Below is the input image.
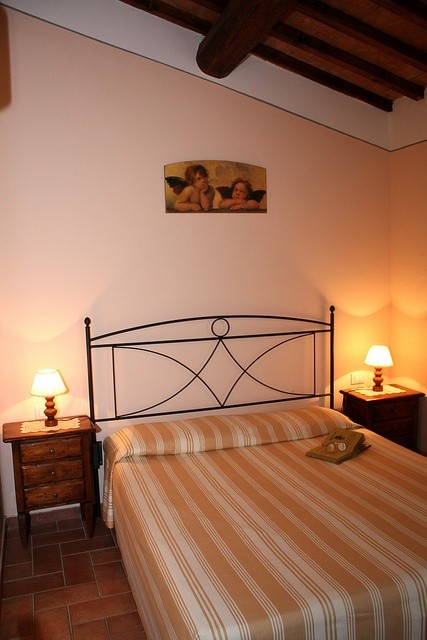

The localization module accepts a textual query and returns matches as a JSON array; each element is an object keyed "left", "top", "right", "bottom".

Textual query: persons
[
  {"left": 164, "top": 164, "right": 215, "bottom": 212},
  {"left": 214, "top": 177, "right": 267, "bottom": 212}
]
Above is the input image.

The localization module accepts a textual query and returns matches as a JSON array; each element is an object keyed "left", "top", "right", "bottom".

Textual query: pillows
[{"left": 124, "top": 403, "right": 353, "bottom": 456}]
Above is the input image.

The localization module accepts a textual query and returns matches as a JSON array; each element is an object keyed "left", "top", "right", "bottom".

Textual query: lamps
[
  {"left": 29, "top": 368, "right": 68, "bottom": 428},
  {"left": 365, "top": 345, "right": 395, "bottom": 392}
]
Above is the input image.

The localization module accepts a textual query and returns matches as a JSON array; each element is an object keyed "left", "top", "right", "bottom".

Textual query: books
[{"left": 303, "top": 427, "right": 369, "bottom": 464}]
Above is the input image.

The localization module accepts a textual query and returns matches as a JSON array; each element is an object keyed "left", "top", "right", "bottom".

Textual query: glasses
[{"left": 321, "top": 442, "right": 347, "bottom": 452}]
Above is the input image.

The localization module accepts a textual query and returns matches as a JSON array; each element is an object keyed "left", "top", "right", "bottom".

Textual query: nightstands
[
  {"left": 339, "top": 384, "right": 425, "bottom": 453},
  {"left": 2, "top": 415, "right": 96, "bottom": 547}
]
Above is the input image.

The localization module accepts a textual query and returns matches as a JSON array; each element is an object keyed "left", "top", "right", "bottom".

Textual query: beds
[{"left": 85, "top": 305, "right": 426, "bottom": 640}]
